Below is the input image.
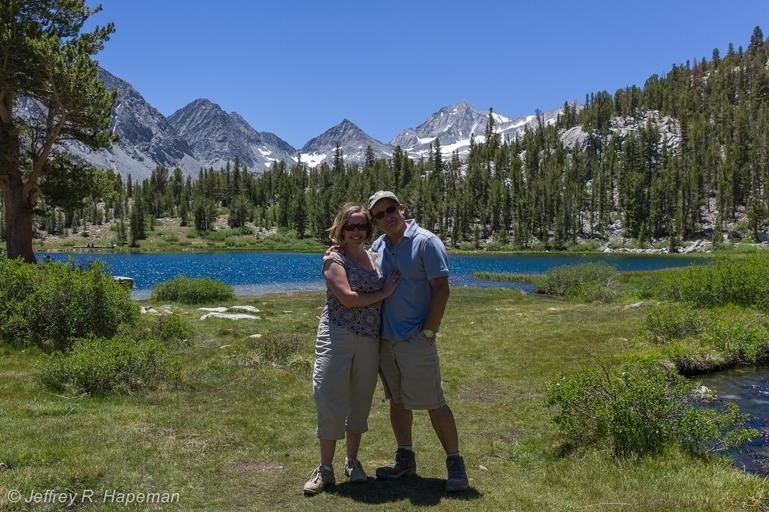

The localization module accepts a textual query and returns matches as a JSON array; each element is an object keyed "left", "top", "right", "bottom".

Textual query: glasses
[
  {"left": 344, "top": 222, "right": 368, "bottom": 232},
  {"left": 371, "top": 203, "right": 398, "bottom": 218}
]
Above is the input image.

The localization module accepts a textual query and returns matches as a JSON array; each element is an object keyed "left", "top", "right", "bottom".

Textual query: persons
[
  {"left": 303, "top": 201, "right": 402, "bottom": 495},
  {"left": 324, "top": 191, "right": 468, "bottom": 492}
]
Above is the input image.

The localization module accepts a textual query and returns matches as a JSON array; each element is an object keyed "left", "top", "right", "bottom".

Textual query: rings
[{"left": 392, "top": 279, "right": 396, "bottom": 283}]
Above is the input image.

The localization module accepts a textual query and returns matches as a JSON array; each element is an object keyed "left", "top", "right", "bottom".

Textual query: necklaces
[{"left": 357, "top": 260, "right": 361, "bottom": 267}]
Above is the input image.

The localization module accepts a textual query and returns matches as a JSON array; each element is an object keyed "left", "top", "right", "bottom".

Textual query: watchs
[{"left": 424, "top": 328, "right": 437, "bottom": 341}]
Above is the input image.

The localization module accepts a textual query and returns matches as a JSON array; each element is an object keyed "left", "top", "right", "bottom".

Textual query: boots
[
  {"left": 444, "top": 454, "right": 470, "bottom": 491},
  {"left": 376, "top": 449, "right": 417, "bottom": 479}
]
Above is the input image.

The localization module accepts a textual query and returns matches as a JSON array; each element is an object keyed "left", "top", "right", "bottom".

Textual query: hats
[{"left": 367, "top": 190, "right": 402, "bottom": 208}]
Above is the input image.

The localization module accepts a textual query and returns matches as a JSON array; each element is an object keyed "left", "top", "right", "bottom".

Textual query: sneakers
[
  {"left": 344, "top": 458, "right": 369, "bottom": 483},
  {"left": 305, "top": 465, "right": 336, "bottom": 494}
]
